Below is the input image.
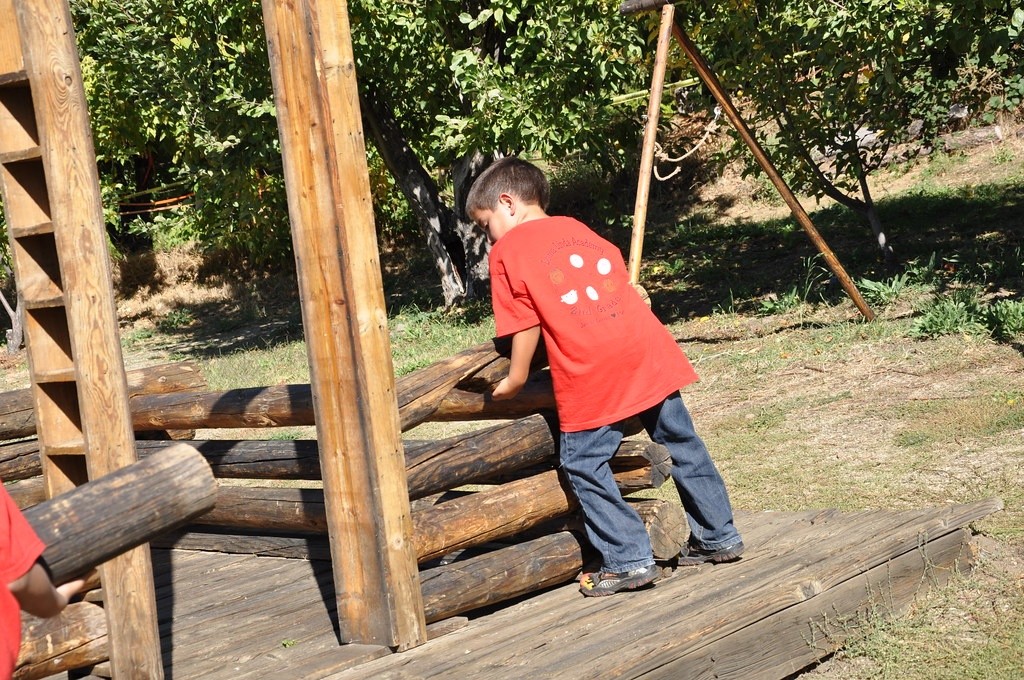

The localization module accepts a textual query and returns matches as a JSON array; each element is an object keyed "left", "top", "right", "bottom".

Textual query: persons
[
  {"left": 463, "top": 156, "right": 744, "bottom": 597},
  {"left": 0, "top": 481, "right": 87, "bottom": 680}
]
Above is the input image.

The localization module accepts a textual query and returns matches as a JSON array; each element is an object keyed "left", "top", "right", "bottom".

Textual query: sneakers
[
  {"left": 678, "top": 541, "right": 745, "bottom": 566},
  {"left": 580, "top": 564, "right": 658, "bottom": 597}
]
